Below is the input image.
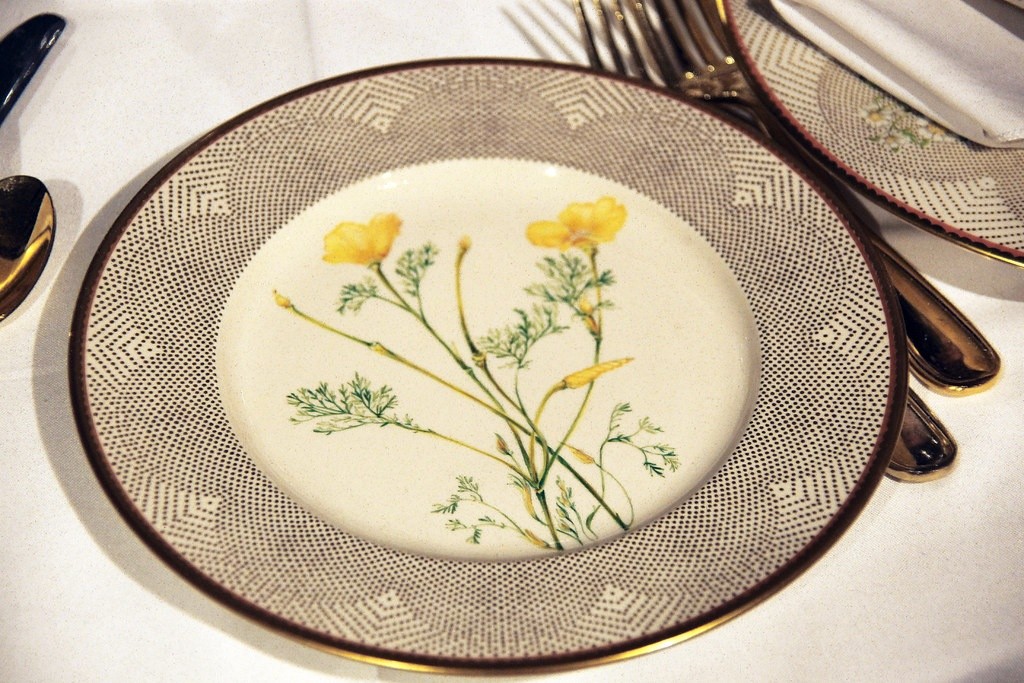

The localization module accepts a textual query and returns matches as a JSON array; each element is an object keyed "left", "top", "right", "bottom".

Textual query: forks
[{"left": 578, "top": 1, "right": 1000, "bottom": 485}]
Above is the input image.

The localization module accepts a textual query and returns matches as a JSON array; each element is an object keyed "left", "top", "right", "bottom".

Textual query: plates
[
  {"left": 722, "top": 3, "right": 1024, "bottom": 266},
  {"left": 65, "top": 58, "right": 912, "bottom": 683}
]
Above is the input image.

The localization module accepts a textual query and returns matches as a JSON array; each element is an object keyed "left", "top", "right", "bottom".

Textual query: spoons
[{"left": 0, "top": 176, "right": 56, "bottom": 323}]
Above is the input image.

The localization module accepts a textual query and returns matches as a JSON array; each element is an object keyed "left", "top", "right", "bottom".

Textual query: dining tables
[{"left": 1, "top": 0, "right": 1019, "bottom": 683}]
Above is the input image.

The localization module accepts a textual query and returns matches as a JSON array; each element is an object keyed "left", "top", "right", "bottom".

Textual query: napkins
[{"left": 770, "top": 0, "right": 1022, "bottom": 150}]
